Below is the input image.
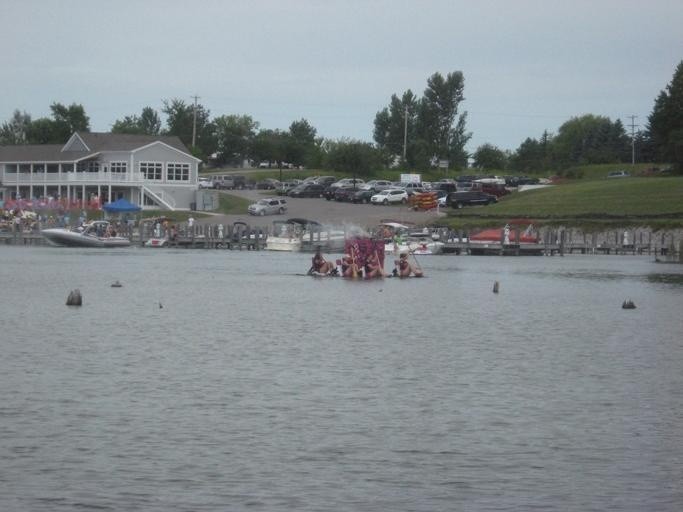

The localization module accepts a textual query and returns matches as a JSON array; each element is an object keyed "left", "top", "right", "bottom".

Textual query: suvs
[{"left": 604, "top": 170, "right": 630, "bottom": 177}]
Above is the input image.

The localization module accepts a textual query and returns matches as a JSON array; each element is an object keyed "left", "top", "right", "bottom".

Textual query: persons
[
  {"left": 0, "top": 193, "right": 196, "bottom": 241},
  {"left": 311, "top": 225, "right": 423, "bottom": 281}
]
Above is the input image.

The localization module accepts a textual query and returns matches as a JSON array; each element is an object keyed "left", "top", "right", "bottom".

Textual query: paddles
[
  {"left": 350, "top": 247, "right": 358, "bottom": 276},
  {"left": 375, "top": 249, "right": 384, "bottom": 276}
]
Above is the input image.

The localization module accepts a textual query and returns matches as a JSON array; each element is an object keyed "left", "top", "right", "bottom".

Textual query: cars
[{"left": 661, "top": 164, "right": 678, "bottom": 172}]
